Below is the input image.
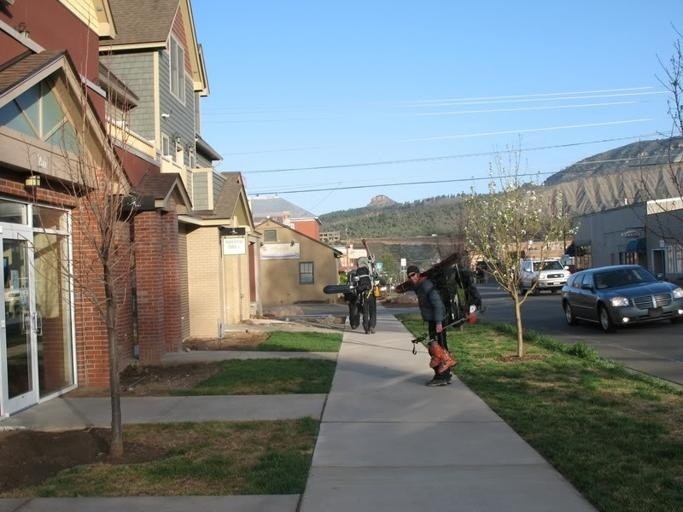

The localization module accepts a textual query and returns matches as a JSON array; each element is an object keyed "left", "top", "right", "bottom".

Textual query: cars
[{"left": 560, "top": 262, "right": 682, "bottom": 333}]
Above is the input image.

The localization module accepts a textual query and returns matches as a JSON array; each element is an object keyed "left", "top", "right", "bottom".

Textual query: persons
[
  {"left": 357, "top": 261, "right": 381, "bottom": 335},
  {"left": 343, "top": 261, "right": 361, "bottom": 330},
  {"left": 406, "top": 264, "right": 453, "bottom": 387}
]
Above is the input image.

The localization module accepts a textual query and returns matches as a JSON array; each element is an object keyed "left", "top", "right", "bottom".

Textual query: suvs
[{"left": 511, "top": 257, "right": 571, "bottom": 294}]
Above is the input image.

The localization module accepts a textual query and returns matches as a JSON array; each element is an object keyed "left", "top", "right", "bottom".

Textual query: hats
[{"left": 407, "top": 266, "right": 420, "bottom": 275}]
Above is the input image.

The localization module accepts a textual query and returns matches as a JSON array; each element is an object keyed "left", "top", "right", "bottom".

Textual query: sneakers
[
  {"left": 425, "top": 377, "right": 452, "bottom": 387},
  {"left": 351, "top": 325, "right": 375, "bottom": 335}
]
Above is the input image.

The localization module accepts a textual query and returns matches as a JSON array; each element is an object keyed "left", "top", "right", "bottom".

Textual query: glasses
[{"left": 408, "top": 272, "right": 416, "bottom": 279}]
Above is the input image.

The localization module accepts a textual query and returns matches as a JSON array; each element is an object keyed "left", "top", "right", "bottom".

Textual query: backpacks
[
  {"left": 427, "top": 263, "right": 483, "bottom": 330},
  {"left": 355, "top": 267, "right": 372, "bottom": 293}
]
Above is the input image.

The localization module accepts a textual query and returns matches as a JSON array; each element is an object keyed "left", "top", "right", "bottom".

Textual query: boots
[
  {"left": 437, "top": 346, "right": 457, "bottom": 375},
  {"left": 427, "top": 339, "right": 448, "bottom": 368}
]
